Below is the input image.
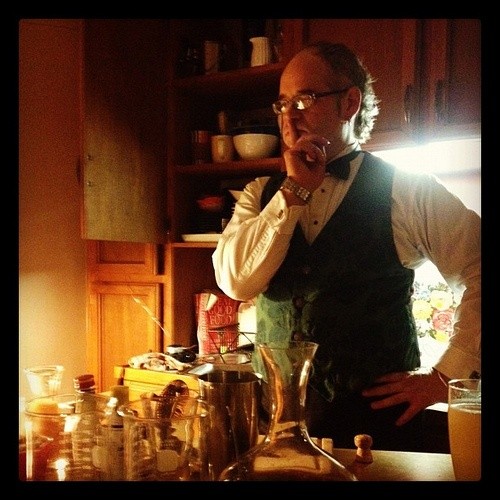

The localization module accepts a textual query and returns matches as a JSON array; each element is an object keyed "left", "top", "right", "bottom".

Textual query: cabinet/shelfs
[
  {"left": 80, "top": 210, "right": 218, "bottom": 422},
  {"left": 76, "top": 16, "right": 304, "bottom": 251},
  {"left": 277, "top": 18, "right": 482, "bottom": 154}
]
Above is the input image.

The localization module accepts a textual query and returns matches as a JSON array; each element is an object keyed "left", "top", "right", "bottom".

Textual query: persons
[{"left": 211, "top": 41, "right": 481, "bottom": 452}]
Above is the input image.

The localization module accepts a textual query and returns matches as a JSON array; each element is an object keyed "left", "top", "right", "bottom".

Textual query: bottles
[
  {"left": 218, "top": 337, "right": 360, "bottom": 479},
  {"left": 249, "top": 33, "right": 274, "bottom": 66}
]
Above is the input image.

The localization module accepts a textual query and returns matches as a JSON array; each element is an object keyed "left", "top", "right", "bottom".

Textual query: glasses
[{"left": 272, "top": 88, "right": 348, "bottom": 115}]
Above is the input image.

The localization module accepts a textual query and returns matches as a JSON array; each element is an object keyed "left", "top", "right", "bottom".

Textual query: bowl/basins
[
  {"left": 196, "top": 194, "right": 224, "bottom": 212},
  {"left": 233, "top": 133, "right": 278, "bottom": 161}
]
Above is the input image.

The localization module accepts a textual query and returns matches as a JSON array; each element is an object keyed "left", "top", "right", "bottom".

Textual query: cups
[
  {"left": 25, "top": 360, "right": 260, "bottom": 481},
  {"left": 210, "top": 134, "right": 233, "bottom": 161},
  {"left": 447, "top": 374, "right": 480, "bottom": 481}
]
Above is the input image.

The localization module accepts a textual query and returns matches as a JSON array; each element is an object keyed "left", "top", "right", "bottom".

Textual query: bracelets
[
  {"left": 281, "top": 177, "right": 313, "bottom": 204},
  {"left": 438, "top": 370, "right": 449, "bottom": 390}
]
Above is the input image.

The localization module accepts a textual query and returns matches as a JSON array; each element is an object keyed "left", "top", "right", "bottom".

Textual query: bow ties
[{"left": 325, "top": 156, "right": 351, "bottom": 180}]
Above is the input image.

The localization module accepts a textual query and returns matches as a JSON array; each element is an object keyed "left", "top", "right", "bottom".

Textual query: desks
[{"left": 19, "top": 435, "right": 457, "bottom": 482}]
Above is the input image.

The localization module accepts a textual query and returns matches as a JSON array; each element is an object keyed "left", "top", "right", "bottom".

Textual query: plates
[{"left": 181, "top": 230, "right": 223, "bottom": 243}]
